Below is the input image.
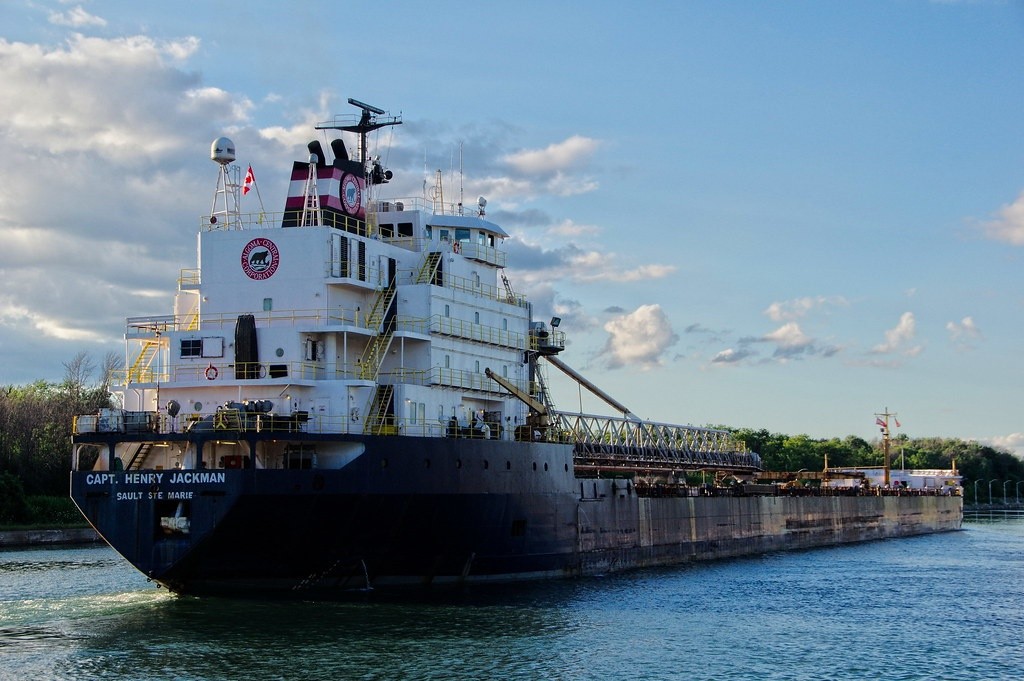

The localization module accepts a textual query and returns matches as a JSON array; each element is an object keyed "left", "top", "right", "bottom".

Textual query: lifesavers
[
  {"left": 453, "top": 242, "right": 459, "bottom": 254},
  {"left": 204, "top": 367, "right": 219, "bottom": 380}
]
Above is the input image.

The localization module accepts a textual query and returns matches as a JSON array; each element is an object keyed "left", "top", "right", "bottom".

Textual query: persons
[{"left": 481, "top": 420, "right": 491, "bottom": 439}]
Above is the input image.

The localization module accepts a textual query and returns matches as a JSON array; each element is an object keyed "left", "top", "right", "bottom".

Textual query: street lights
[{"left": 973, "top": 480, "right": 1024, "bottom": 505}]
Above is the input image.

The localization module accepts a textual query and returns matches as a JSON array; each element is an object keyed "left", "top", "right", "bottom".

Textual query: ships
[{"left": 69, "top": 95, "right": 963, "bottom": 607}]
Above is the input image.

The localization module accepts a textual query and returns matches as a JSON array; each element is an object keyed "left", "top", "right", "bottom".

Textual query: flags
[
  {"left": 876, "top": 418, "right": 887, "bottom": 429},
  {"left": 243, "top": 167, "right": 255, "bottom": 196}
]
